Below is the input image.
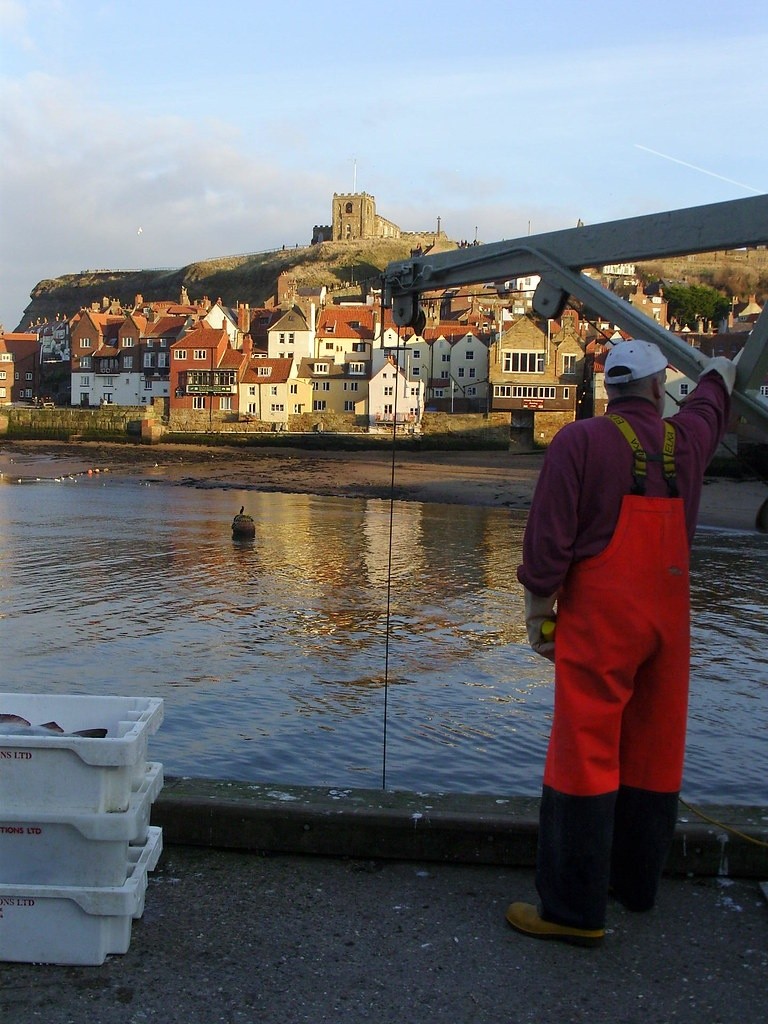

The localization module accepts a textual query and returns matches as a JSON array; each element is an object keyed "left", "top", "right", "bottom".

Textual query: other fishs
[{"left": 0, "top": 712, "right": 108, "bottom": 744}]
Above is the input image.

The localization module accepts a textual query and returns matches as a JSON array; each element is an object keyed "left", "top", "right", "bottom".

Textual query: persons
[{"left": 506, "top": 339, "right": 737, "bottom": 947}]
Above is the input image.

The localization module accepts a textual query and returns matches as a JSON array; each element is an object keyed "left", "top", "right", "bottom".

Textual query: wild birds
[{"left": 9, "top": 456, "right": 159, "bottom": 486}]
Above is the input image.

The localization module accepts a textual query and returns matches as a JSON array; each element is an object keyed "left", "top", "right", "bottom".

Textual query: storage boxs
[{"left": 0, "top": 692, "right": 164, "bottom": 968}]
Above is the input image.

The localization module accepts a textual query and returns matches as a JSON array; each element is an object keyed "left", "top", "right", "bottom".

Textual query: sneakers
[{"left": 504, "top": 901, "right": 605, "bottom": 950}]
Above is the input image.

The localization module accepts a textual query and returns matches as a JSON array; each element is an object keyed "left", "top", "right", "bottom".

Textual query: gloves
[
  {"left": 524, "top": 584, "right": 560, "bottom": 665},
  {"left": 699, "top": 356, "right": 736, "bottom": 396}
]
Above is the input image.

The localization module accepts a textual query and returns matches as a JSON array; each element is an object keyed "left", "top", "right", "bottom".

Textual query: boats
[{"left": 233, "top": 504, "right": 256, "bottom": 538}]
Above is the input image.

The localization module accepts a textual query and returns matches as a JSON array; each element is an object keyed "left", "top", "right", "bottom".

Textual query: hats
[{"left": 604, "top": 339, "right": 678, "bottom": 386}]
